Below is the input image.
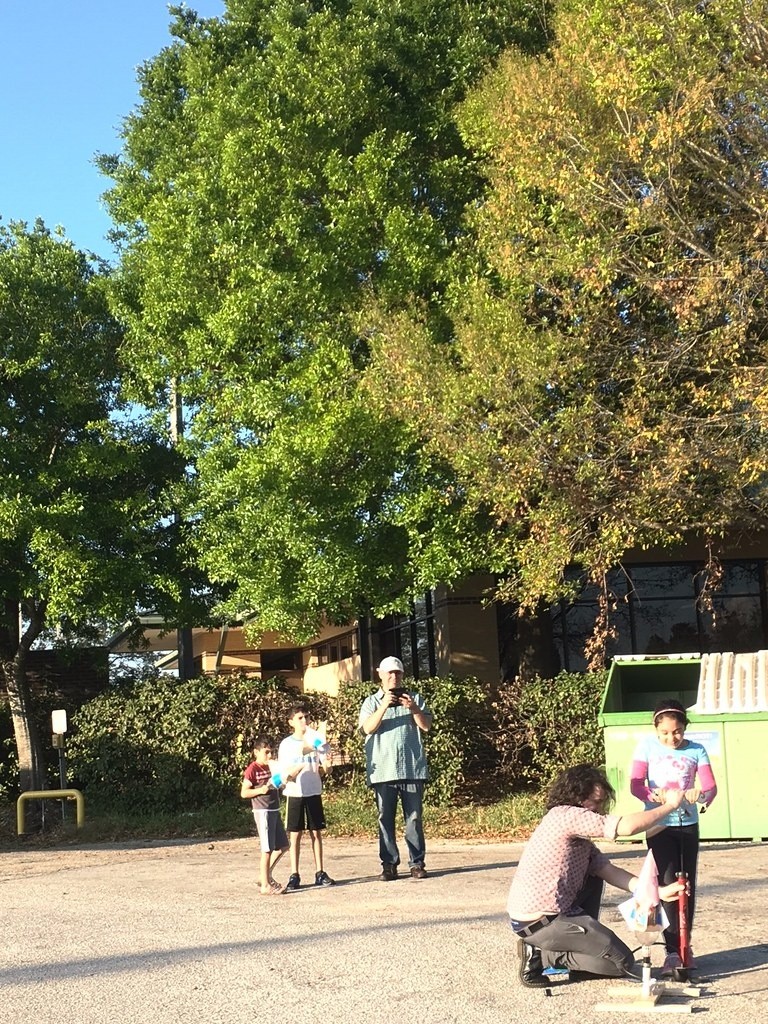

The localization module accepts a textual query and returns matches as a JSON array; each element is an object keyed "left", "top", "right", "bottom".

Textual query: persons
[
  {"left": 630, "top": 703, "right": 718, "bottom": 975},
  {"left": 357, "top": 656, "right": 431, "bottom": 880},
  {"left": 241, "top": 736, "right": 289, "bottom": 894},
  {"left": 277, "top": 703, "right": 335, "bottom": 890},
  {"left": 507, "top": 764, "right": 683, "bottom": 988}
]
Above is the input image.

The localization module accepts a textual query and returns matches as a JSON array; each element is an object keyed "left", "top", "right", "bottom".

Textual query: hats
[{"left": 379, "top": 656, "right": 405, "bottom": 673}]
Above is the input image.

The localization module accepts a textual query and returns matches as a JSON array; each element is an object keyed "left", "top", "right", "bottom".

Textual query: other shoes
[
  {"left": 316, "top": 871, "right": 336, "bottom": 886},
  {"left": 286, "top": 876, "right": 299, "bottom": 889},
  {"left": 411, "top": 868, "right": 427, "bottom": 877},
  {"left": 659, "top": 951, "right": 683, "bottom": 976},
  {"left": 517, "top": 938, "right": 550, "bottom": 988},
  {"left": 568, "top": 970, "right": 627, "bottom": 982},
  {"left": 379, "top": 870, "right": 398, "bottom": 881},
  {"left": 680, "top": 947, "right": 698, "bottom": 970},
  {"left": 259, "top": 880, "right": 286, "bottom": 894}
]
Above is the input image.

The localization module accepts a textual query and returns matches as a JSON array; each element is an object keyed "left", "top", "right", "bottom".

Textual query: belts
[{"left": 516, "top": 915, "right": 558, "bottom": 938}]
